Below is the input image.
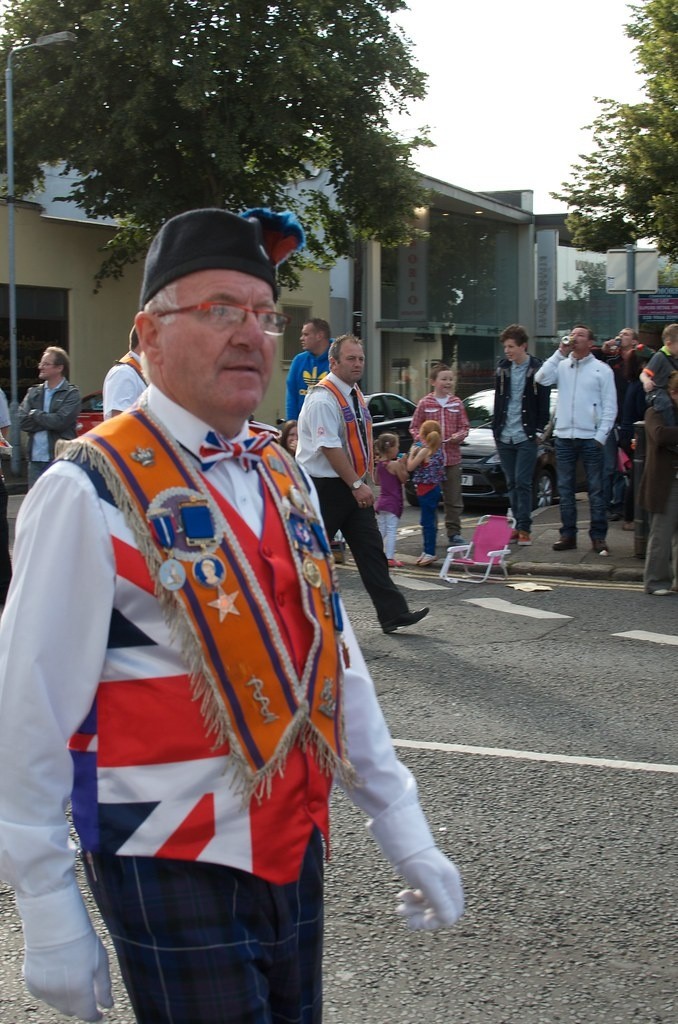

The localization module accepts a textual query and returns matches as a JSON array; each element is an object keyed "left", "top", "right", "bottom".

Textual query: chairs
[
  {"left": 437, "top": 514, "right": 517, "bottom": 584},
  {"left": 370, "top": 404, "right": 383, "bottom": 416}
]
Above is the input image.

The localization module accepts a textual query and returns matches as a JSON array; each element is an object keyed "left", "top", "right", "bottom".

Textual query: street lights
[{"left": 5, "top": 31, "right": 77, "bottom": 478}]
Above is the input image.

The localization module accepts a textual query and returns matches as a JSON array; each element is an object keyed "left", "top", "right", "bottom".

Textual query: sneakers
[
  {"left": 449, "top": 534, "right": 466, "bottom": 545},
  {"left": 517, "top": 531, "right": 533, "bottom": 546},
  {"left": 624, "top": 521, "right": 637, "bottom": 531},
  {"left": 553, "top": 536, "right": 577, "bottom": 550},
  {"left": 593, "top": 539, "right": 608, "bottom": 553},
  {"left": 509, "top": 529, "right": 517, "bottom": 544}
]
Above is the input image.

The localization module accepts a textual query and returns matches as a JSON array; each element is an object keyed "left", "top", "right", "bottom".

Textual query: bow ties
[{"left": 199, "top": 431, "right": 271, "bottom": 473}]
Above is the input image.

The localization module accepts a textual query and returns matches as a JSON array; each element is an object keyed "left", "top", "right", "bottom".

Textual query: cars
[
  {"left": 77, "top": 387, "right": 105, "bottom": 439},
  {"left": 363, "top": 392, "right": 418, "bottom": 456},
  {"left": 405, "top": 387, "right": 582, "bottom": 511}
]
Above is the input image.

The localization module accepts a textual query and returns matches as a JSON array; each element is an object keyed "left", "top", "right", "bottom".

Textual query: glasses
[
  {"left": 39, "top": 362, "right": 61, "bottom": 368},
  {"left": 157, "top": 302, "right": 288, "bottom": 336}
]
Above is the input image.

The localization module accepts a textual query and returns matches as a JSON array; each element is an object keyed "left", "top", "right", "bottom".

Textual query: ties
[{"left": 350, "top": 389, "right": 368, "bottom": 456}]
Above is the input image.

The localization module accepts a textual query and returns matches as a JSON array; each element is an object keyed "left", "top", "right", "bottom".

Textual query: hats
[{"left": 138, "top": 208, "right": 302, "bottom": 309}]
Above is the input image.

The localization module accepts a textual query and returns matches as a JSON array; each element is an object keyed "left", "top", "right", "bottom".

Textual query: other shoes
[
  {"left": 651, "top": 588, "right": 676, "bottom": 595},
  {"left": 387, "top": 559, "right": 402, "bottom": 566},
  {"left": 416, "top": 553, "right": 425, "bottom": 564},
  {"left": 419, "top": 554, "right": 438, "bottom": 565}
]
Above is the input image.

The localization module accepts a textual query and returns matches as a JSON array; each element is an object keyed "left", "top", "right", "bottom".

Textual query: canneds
[{"left": 561, "top": 336, "right": 575, "bottom": 345}]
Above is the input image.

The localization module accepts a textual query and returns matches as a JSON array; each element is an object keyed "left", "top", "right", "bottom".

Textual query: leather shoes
[{"left": 381, "top": 607, "right": 429, "bottom": 634}]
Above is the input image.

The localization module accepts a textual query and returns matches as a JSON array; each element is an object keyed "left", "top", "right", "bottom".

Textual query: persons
[
  {"left": 102, "top": 325, "right": 150, "bottom": 420},
  {"left": 294, "top": 334, "right": 430, "bottom": 633},
  {"left": 280, "top": 419, "right": 298, "bottom": 456},
  {"left": 286, "top": 318, "right": 337, "bottom": 421},
  {"left": 0, "top": 208, "right": 463, "bottom": 1024},
  {"left": 409, "top": 364, "right": 469, "bottom": 545},
  {"left": 534, "top": 325, "right": 618, "bottom": 556},
  {"left": 17, "top": 347, "right": 81, "bottom": 490},
  {"left": 407, "top": 420, "right": 447, "bottom": 565},
  {"left": 376, "top": 434, "right": 407, "bottom": 567},
  {"left": 0, "top": 389, "right": 11, "bottom": 438},
  {"left": 590, "top": 324, "right": 678, "bottom": 595},
  {"left": 492, "top": 324, "right": 550, "bottom": 545}
]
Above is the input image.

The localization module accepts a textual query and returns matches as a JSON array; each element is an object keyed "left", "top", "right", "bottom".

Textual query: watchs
[{"left": 350, "top": 479, "right": 361, "bottom": 490}]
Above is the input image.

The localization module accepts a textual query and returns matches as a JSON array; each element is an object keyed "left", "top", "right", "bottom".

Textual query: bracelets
[
  {"left": 29, "top": 410, "right": 35, "bottom": 415},
  {"left": 536, "top": 433, "right": 543, "bottom": 439}
]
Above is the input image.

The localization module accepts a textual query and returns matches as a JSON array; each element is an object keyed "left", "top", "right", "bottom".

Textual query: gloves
[
  {"left": 394, "top": 848, "right": 463, "bottom": 932},
  {"left": 16, "top": 886, "right": 114, "bottom": 1021}
]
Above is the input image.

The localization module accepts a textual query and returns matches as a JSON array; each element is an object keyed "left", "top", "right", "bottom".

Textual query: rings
[{"left": 363, "top": 502, "right": 366, "bottom": 504}]
